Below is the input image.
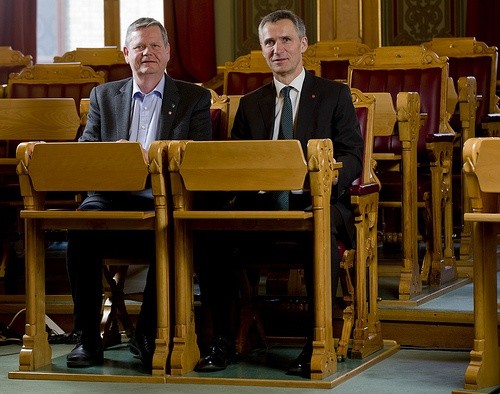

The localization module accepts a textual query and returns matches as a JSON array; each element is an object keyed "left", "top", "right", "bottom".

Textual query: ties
[{"left": 273, "top": 86, "right": 294, "bottom": 211}]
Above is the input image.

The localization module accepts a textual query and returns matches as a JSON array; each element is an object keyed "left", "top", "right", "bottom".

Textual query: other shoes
[
  {"left": 199, "top": 338, "right": 241, "bottom": 372},
  {"left": 129, "top": 333, "right": 155, "bottom": 366},
  {"left": 296, "top": 346, "right": 311, "bottom": 375},
  {"left": 67, "top": 338, "right": 104, "bottom": 368}
]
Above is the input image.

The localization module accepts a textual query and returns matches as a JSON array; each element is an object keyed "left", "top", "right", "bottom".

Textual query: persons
[
  {"left": 194, "top": 11, "right": 364, "bottom": 373},
  {"left": 66, "top": 18, "right": 211, "bottom": 370}
]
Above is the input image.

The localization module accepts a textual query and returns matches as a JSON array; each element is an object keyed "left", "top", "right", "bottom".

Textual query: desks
[{"left": 10, "top": 138, "right": 500, "bottom": 394}]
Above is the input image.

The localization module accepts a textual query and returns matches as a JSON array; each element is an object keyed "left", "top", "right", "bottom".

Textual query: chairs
[{"left": 1, "top": 37, "right": 500, "bottom": 356}]
